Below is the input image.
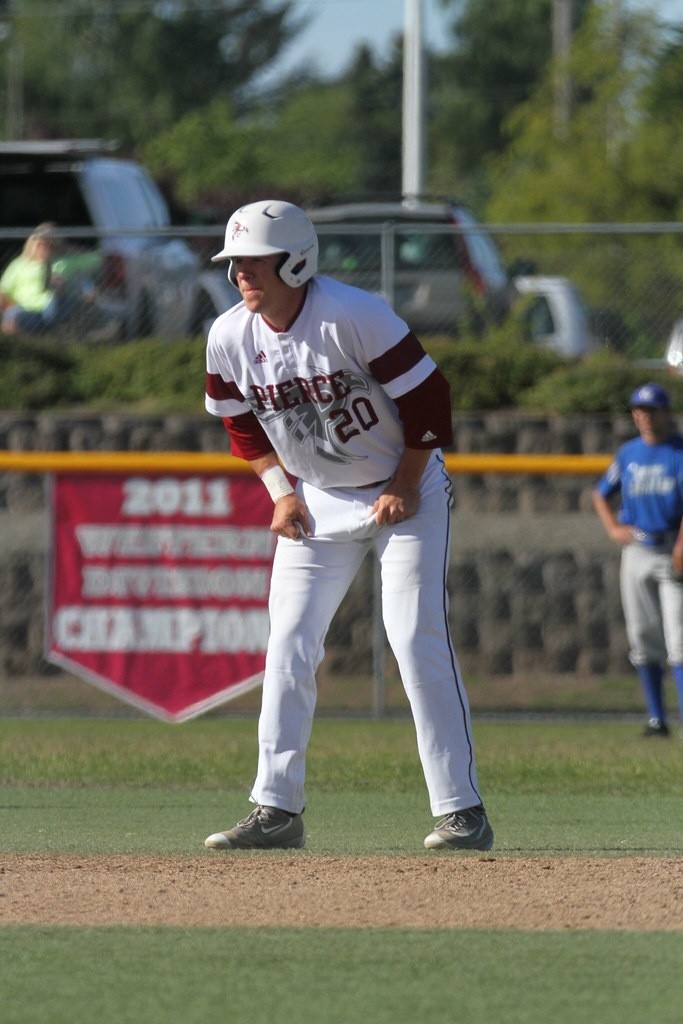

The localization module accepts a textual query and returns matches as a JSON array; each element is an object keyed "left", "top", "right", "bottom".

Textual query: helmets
[
  {"left": 630, "top": 384, "right": 670, "bottom": 410},
  {"left": 211, "top": 199, "right": 318, "bottom": 289}
]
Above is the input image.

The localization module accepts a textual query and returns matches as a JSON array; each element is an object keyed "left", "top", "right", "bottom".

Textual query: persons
[
  {"left": 202, "top": 201, "right": 494, "bottom": 851},
  {"left": 592, "top": 384, "right": 683, "bottom": 737},
  {"left": 0, "top": 222, "right": 104, "bottom": 336}
]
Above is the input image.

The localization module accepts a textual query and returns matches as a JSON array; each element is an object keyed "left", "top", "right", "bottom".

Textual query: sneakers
[
  {"left": 204, "top": 798, "right": 306, "bottom": 848},
  {"left": 424, "top": 803, "right": 494, "bottom": 850}
]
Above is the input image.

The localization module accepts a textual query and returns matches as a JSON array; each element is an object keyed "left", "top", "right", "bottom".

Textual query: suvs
[{"left": 0, "top": 135, "right": 208, "bottom": 347}]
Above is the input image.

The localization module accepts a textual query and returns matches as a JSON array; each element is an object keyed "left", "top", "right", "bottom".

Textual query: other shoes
[{"left": 642, "top": 723, "right": 669, "bottom": 738}]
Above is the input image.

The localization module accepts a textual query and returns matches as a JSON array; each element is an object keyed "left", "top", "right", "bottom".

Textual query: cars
[{"left": 183, "top": 193, "right": 605, "bottom": 364}]
